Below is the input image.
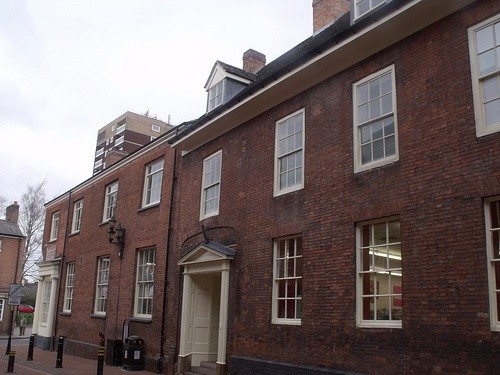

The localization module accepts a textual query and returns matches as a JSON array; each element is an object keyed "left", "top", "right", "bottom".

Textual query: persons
[{"left": 19, "top": 314, "right": 27, "bottom": 336}]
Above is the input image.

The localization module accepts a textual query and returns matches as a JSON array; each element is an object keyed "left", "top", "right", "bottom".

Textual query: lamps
[{"left": 106, "top": 214, "right": 124, "bottom": 246}]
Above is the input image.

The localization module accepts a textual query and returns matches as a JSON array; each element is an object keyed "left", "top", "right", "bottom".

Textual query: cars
[{"left": 17, "top": 304, "right": 35, "bottom": 313}]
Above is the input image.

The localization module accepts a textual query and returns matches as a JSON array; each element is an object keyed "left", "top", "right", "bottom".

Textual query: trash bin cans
[
  {"left": 105, "top": 339, "right": 125, "bottom": 366},
  {"left": 123, "top": 336, "right": 146, "bottom": 372}
]
